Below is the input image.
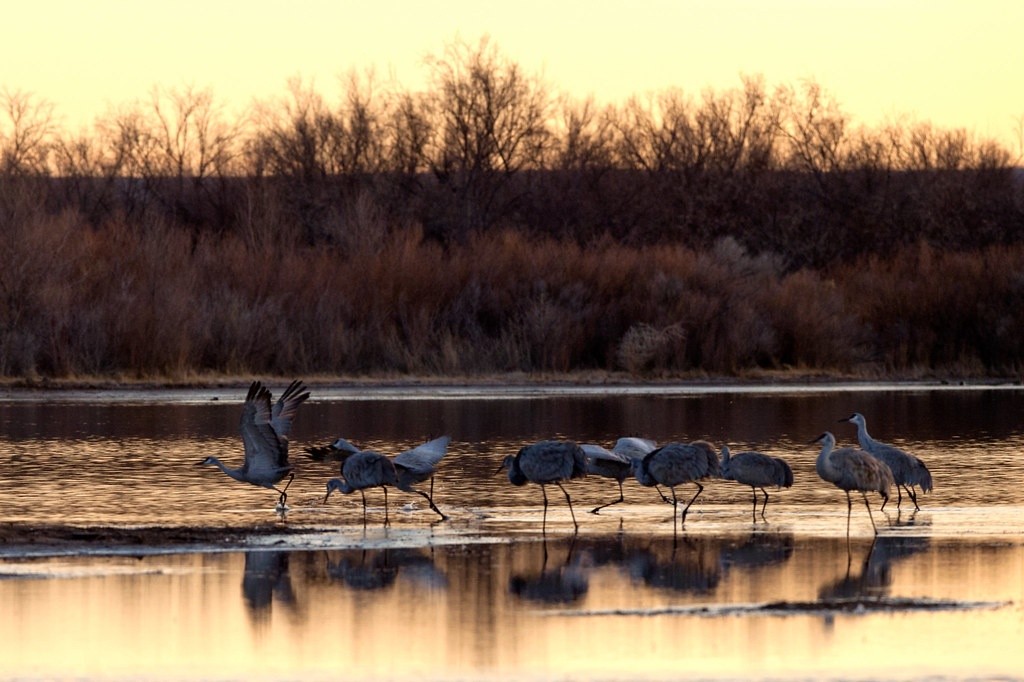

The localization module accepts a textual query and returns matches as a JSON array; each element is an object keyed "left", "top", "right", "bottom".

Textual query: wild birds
[
  {"left": 508, "top": 536, "right": 794, "bottom": 608},
  {"left": 494, "top": 441, "right": 589, "bottom": 529},
  {"left": 629, "top": 440, "right": 720, "bottom": 532},
  {"left": 720, "top": 445, "right": 793, "bottom": 525},
  {"left": 578, "top": 437, "right": 662, "bottom": 514},
  {"left": 303, "top": 433, "right": 454, "bottom": 524},
  {"left": 323, "top": 452, "right": 400, "bottom": 529},
  {"left": 241, "top": 547, "right": 449, "bottom": 617},
  {"left": 806, "top": 431, "right": 895, "bottom": 536},
  {"left": 819, "top": 537, "right": 932, "bottom": 628},
  {"left": 839, "top": 413, "right": 933, "bottom": 510},
  {"left": 193, "top": 379, "right": 311, "bottom": 523}
]
